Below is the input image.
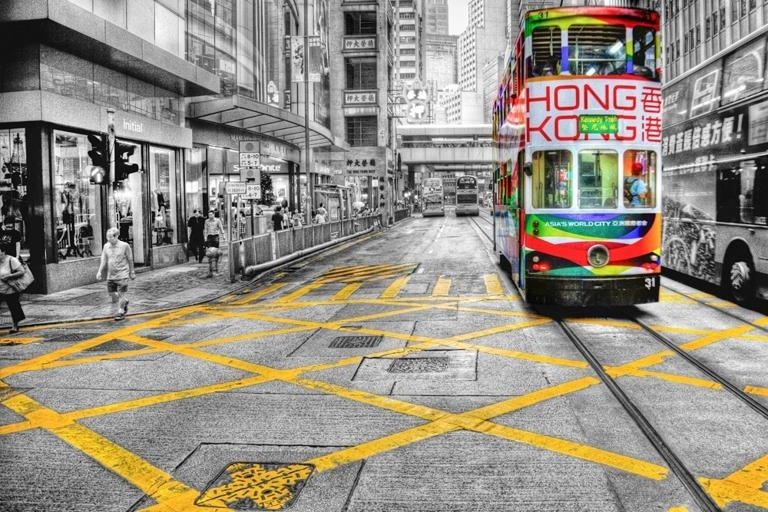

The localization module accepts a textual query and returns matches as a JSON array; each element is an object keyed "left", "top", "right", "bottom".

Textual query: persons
[
  {"left": 214, "top": 208, "right": 219, "bottom": 217},
  {"left": 231, "top": 202, "right": 237, "bottom": 233},
  {"left": 533, "top": 46, "right": 654, "bottom": 80},
  {"left": 271, "top": 205, "right": 284, "bottom": 231},
  {"left": 186, "top": 209, "right": 206, "bottom": 263},
  {"left": 236, "top": 210, "right": 246, "bottom": 245},
  {"left": 0, "top": 243, "right": 26, "bottom": 335},
  {"left": 281, "top": 196, "right": 288, "bottom": 212},
  {"left": 95, "top": 228, "right": 135, "bottom": 320},
  {"left": 623, "top": 163, "right": 649, "bottom": 208},
  {"left": 311, "top": 210, "right": 326, "bottom": 223},
  {"left": 281, "top": 210, "right": 289, "bottom": 229},
  {"left": 318, "top": 202, "right": 328, "bottom": 221},
  {"left": 243, "top": 202, "right": 247, "bottom": 214},
  {"left": 203, "top": 210, "right": 225, "bottom": 248}
]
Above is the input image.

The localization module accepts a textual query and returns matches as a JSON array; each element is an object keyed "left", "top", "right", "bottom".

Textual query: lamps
[{"left": 5, "top": 133, "right": 27, "bottom": 174}]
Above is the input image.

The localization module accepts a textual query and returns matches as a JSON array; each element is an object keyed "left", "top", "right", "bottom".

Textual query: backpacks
[{"left": 624, "top": 178, "right": 638, "bottom": 202}]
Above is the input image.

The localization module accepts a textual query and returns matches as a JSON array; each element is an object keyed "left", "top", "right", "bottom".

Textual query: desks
[{"left": 55, "top": 222, "right": 89, "bottom": 247}]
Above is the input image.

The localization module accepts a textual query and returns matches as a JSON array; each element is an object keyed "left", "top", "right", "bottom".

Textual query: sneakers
[
  {"left": 9, "top": 326, "right": 18, "bottom": 334},
  {"left": 115, "top": 300, "right": 129, "bottom": 320}
]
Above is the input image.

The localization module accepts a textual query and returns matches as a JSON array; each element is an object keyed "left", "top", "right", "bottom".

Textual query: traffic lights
[
  {"left": 115, "top": 140, "right": 138, "bottom": 180},
  {"left": 88, "top": 132, "right": 110, "bottom": 185}
]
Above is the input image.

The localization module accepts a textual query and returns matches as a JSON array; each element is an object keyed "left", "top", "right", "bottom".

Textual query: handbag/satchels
[{"left": 6, "top": 264, "right": 34, "bottom": 292}]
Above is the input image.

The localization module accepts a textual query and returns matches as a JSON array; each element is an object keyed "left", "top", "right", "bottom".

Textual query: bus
[
  {"left": 454, "top": 175, "right": 479, "bottom": 217},
  {"left": 490, "top": 5, "right": 664, "bottom": 310},
  {"left": 657, "top": 25, "right": 767, "bottom": 301},
  {"left": 420, "top": 177, "right": 445, "bottom": 217}
]
